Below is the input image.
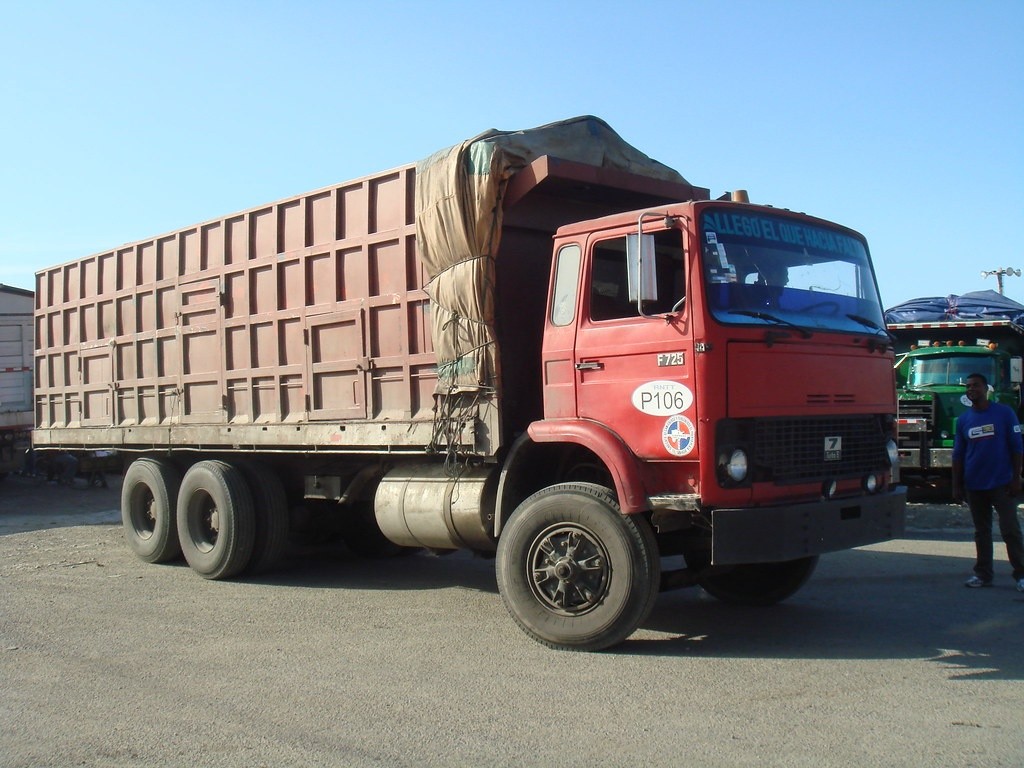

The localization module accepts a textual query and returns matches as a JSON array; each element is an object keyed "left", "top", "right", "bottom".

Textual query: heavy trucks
[
  {"left": 883, "top": 289, "right": 1023, "bottom": 503},
  {"left": 32, "top": 115, "right": 905, "bottom": 654}
]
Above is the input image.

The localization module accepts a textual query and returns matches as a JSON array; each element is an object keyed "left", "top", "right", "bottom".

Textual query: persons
[
  {"left": 952, "top": 374, "right": 1024, "bottom": 592},
  {"left": 746, "top": 263, "right": 789, "bottom": 307}
]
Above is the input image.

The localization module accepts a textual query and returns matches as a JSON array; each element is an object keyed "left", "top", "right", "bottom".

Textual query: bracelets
[{"left": 1015, "top": 472, "right": 1022, "bottom": 480}]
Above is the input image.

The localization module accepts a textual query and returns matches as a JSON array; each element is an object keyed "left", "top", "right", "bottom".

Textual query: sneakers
[
  {"left": 965, "top": 576, "right": 983, "bottom": 587},
  {"left": 1016, "top": 578, "right": 1024, "bottom": 591}
]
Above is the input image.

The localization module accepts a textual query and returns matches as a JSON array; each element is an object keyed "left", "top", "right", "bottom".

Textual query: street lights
[{"left": 980, "top": 267, "right": 1021, "bottom": 295}]
